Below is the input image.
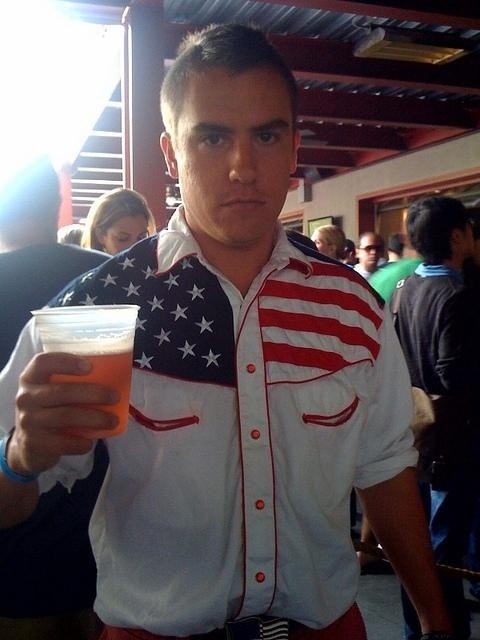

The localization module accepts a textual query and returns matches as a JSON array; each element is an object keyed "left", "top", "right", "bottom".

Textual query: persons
[
  {"left": 1, "top": 23, "right": 448, "bottom": 639},
  {"left": 314, "top": 196, "right": 478, "bottom": 640},
  {"left": 0, "top": 157, "right": 157, "bottom": 639}
]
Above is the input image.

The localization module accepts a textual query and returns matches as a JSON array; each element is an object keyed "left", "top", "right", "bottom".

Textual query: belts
[{"left": 190, "top": 613, "right": 308, "bottom": 640}]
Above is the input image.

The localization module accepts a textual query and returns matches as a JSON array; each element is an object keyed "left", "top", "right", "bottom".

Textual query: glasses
[{"left": 357, "top": 245, "right": 383, "bottom": 252}]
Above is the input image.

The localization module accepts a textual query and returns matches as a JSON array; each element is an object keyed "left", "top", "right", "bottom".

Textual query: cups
[{"left": 30, "top": 304, "right": 141, "bottom": 437}]
